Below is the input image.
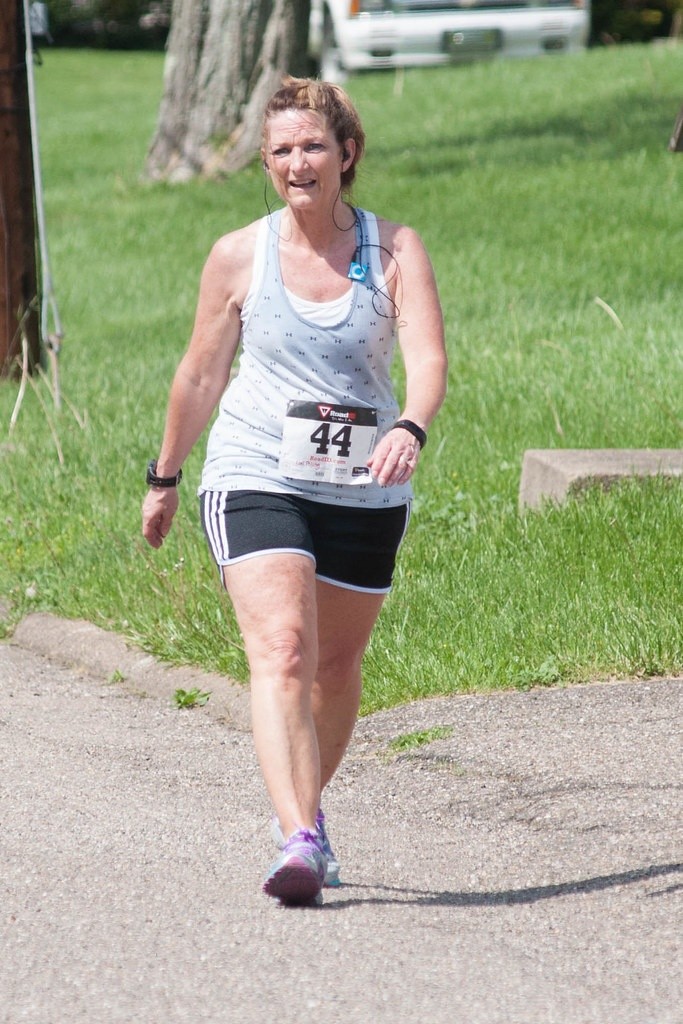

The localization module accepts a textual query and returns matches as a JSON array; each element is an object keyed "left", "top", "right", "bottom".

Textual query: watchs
[{"left": 145, "top": 460, "right": 183, "bottom": 487}]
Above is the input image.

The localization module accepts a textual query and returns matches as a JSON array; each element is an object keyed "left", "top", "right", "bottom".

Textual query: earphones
[
  {"left": 262, "top": 159, "right": 270, "bottom": 170},
  {"left": 341, "top": 147, "right": 350, "bottom": 163}
]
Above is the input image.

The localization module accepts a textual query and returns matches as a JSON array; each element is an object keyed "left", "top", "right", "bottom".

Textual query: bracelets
[{"left": 392, "top": 419, "right": 427, "bottom": 450}]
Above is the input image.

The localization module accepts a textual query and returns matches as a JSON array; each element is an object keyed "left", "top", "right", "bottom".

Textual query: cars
[{"left": 325, "top": 0, "right": 590, "bottom": 76}]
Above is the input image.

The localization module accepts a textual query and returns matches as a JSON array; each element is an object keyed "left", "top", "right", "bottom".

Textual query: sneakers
[
  {"left": 262, "top": 830, "right": 329, "bottom": 908},
  {"left": 269, "top": 807, "right": 342, "bottom": 889}
]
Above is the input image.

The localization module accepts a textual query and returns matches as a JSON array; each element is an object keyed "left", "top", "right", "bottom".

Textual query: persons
[{"left": 141, "top": 74, "right": 447, "bottom": 903}]
